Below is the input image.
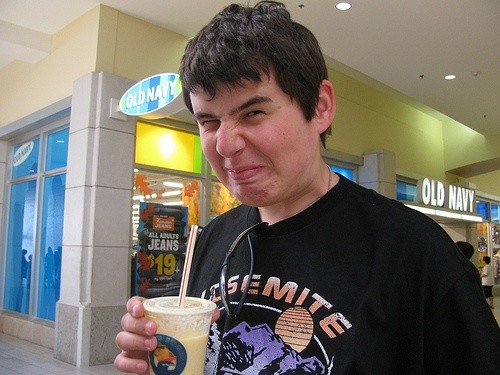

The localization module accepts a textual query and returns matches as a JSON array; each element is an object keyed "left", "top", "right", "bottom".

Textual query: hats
[{"left": 480, "top": 237, "right": 485, "bottom": 240}]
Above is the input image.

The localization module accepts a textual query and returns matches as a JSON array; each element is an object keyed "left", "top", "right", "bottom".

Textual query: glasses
[{"left": 219, "top": 221, "right": 269, "bottom": 321}]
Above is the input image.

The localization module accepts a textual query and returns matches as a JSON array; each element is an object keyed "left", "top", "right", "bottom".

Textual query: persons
[
  {"left": 20, "top": 247, "right": 62, "bottom": 301},
  {"left": 455, "top": 241, "right": 494, "bottom": 310},
  {"left": 114, "top": 0, "right": 500, "bottom": 375}
]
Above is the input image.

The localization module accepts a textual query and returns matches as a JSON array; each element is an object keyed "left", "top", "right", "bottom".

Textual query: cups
[{"left": 143, "top": 296, "right": 217, "bottom": 375}]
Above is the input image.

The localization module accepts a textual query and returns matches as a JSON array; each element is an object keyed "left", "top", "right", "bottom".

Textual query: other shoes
[{"left": 489, "top": 305, "right": 494, "bottom": 309}]
